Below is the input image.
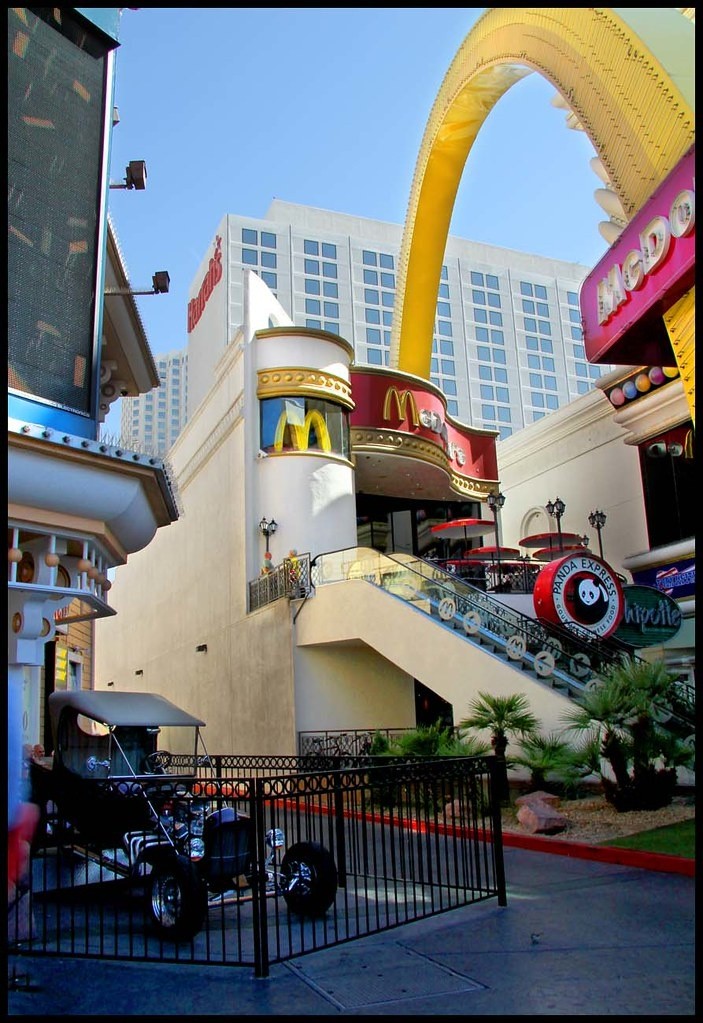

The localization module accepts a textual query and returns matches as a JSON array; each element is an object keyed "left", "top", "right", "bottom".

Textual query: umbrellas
[{"left": 431, "top": 519, "right": 592, "bottom": 590}]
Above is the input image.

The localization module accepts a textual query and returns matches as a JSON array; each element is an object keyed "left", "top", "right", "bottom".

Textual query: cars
[{"left": 29, "top": 688, "right": 338, "bottom": 942}]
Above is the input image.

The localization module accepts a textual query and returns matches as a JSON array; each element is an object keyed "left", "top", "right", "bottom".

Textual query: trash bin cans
[{"left": 447, "top": 560, "right": 486, "bottom": 595}]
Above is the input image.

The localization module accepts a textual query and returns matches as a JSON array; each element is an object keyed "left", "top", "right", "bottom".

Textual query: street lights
[
  {"left": 485, "top": 492, "right": 506, "bottom": 588},
  {"left": 545, "top": 497, "right": 566, "bottom": 557},
  {"left": 587, "top": 508, "right": 606, "bottom": 560},
  {"left": 259, "top": 516, "right": 277, "bottom": 554}
]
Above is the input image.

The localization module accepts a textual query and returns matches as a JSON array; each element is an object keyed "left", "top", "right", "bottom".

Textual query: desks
[
  {"left": 485, "top": 564, "right": 541, "bottom": 593},
  {"left": 440, "top": 560, "right": 488, "bottom": 592}
]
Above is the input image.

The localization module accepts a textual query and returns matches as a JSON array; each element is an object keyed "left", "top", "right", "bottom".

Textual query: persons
[
  {"left": 22, "top": 744, "right": 45, "bottom": 777},
  {"left": 260, "top": 549, "right": 301, "bottom": 590}
]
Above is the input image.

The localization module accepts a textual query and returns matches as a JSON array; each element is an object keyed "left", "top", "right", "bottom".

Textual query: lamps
[
  {"left": 109, "top": 160, "right": 147, "bottom": 191},
  {"left": 135, "top": 670, "right": 143, "bottom": 675},
  {"left": 104, "top": 271, "right": 170, "bottom": 296},
  {"left": 107, "top": 682, "right": 113, "bottom": 686},
  {"left": 196, "top": 644, "right": 207, "bottom": 652}
]
